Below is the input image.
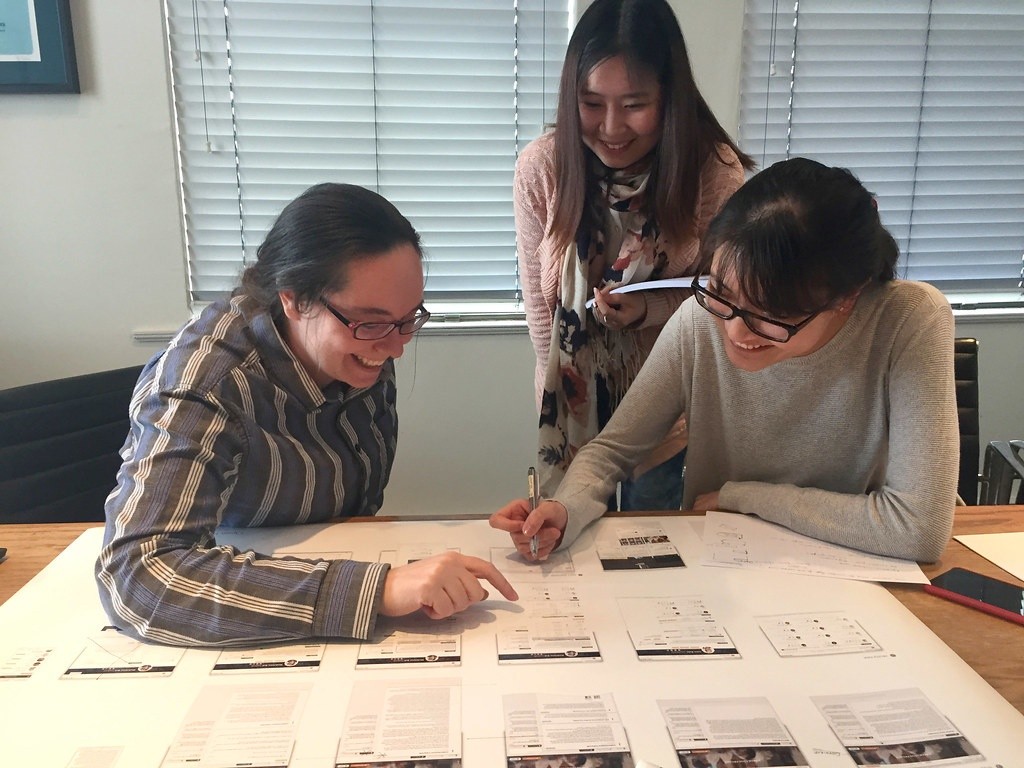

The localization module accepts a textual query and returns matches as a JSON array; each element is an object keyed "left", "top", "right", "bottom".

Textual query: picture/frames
[{"left": 0, "top": 0, "right": 80, "bottom": 95}]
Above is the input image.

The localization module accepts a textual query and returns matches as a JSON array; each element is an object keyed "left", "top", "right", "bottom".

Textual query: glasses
[
  {"left": 316, "top": 296, "right": 431, "bottom": 341},
  {"left": 691, "top": 253, "right": 828, "bottom": 343}
]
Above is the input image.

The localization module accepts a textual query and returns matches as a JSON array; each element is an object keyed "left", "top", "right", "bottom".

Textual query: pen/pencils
[{"left": 527, "top": 467, "right": 539, "bottom": 559}]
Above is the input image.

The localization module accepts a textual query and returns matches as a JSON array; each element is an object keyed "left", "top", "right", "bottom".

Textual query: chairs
[
  {"left": 0, "top": 366, "right": 144, "bottom": 525},
  {"left": 954, "top": 339, "right": 1024, "bottom": 506}
]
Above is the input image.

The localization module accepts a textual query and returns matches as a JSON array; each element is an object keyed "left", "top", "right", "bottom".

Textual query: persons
[
  {"left": 95, "top": 182, "right": 520, "bottom": 646},
  {"left": 513, "top": 1, "right": 756, "bottom": 510},
  {"left": 489, "top": 158, "right": 959, "bottom": 562}
]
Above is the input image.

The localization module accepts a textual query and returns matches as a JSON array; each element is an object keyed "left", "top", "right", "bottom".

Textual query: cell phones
[{"left": 923, "top": 566, "right": 1024, "bottom": 626}]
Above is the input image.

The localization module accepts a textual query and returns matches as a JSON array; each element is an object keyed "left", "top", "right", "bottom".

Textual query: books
[{"left": 585, "top": 276, "right": 710, "bottom": 309}]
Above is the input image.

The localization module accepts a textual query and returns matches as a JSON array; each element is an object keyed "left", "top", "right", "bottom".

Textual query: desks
[{"left": 0, "top": 506, "right": 1024, "bottom": 768}]
